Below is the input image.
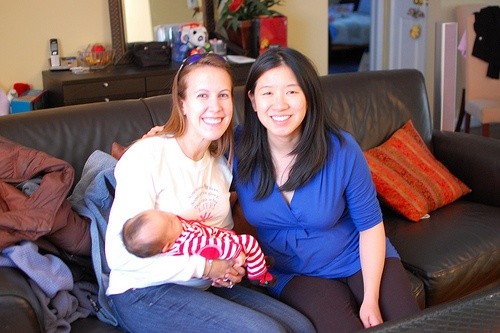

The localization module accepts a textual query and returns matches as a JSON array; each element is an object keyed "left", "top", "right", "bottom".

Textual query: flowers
[{"left": 219, "top": 0, "right": 282, "bottom": 32}]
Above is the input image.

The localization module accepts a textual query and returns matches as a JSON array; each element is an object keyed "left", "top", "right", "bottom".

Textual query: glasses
[{"left": 179, "top": 53, "right": 229, "bottom": 65}]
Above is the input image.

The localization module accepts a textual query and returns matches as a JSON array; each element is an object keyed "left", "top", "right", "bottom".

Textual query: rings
[
  {"left": 227, "top": 281, "right": 234, "bottom": 288},
  {"left": 223, "top": 273, "right": 229, "bottom": 282}
]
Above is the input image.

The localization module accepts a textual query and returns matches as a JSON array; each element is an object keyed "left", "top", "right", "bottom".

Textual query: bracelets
[{"left": 206, "top": 260, "right": 213, "bottom": 277}]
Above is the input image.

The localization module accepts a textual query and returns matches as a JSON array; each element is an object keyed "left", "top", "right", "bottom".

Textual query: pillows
[{"left": 362, "top": 118, "right": 473, "bottom": 221}]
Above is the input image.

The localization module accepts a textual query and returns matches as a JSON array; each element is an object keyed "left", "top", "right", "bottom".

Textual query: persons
[
  {"left": 105, "top": 53, "right": 316, "bottom": 333},
  {"left": 224, "top": 48, "right": 419, "bottom": 333},
  {"left": 122, "top": 209, "right": 278, "bottom": 288}
]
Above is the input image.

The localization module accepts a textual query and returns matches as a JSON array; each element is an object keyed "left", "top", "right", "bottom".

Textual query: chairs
[{"left": 462, "top": 15, "right": 500, "bottom": 139}]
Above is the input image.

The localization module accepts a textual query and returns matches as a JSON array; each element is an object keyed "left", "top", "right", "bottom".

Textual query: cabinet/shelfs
[{"left": 42, "top": 68, "right": 187, "bottom": 108}]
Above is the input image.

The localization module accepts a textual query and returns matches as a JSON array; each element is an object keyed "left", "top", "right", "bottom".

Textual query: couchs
[{"left": 0, "top": 68, "right": 500, "bottom": 333}]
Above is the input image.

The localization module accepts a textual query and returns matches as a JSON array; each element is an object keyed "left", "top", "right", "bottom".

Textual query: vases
[{"left": 225, "top": 21, "right": 255, "bottom": 52}]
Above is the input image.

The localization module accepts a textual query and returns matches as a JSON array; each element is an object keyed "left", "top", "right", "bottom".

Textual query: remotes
[{"left": 50, "top": 39, "right": 58, "bottom": 55}]
